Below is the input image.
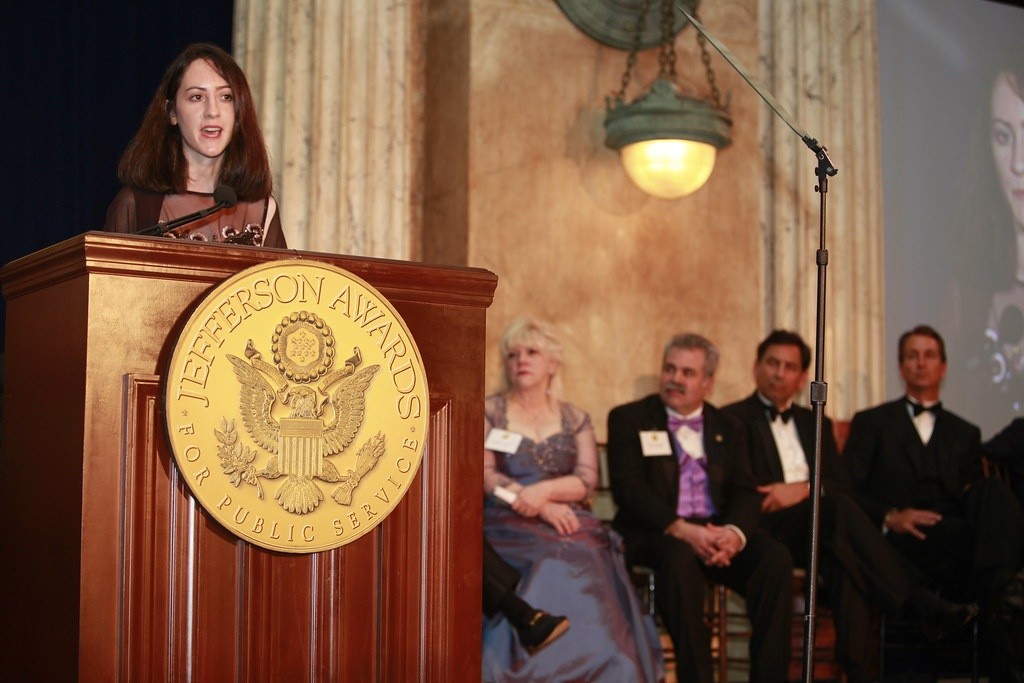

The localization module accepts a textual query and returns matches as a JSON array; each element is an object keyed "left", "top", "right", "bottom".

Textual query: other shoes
[{"left": 518, "top": 609, "right": 569, "bottom": 657}]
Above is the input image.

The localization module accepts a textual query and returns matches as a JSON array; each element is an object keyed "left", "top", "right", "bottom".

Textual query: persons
[
  {"left": 608, "top": 333, "right": 794, "bottom": 683},
  {"left": 721, "top": 330, "right": 900, "bottom": 683},
  {"left": 477, "top": 317, "right": 664, "bottom": 683},
  {"left": 930, "top": 35, "right": 1023, "bottom": 395},
  {"left": 102, "top": 44, "right": 286, "bottom": 250},
  {"left": 838, "top": 325, "right": 1024, "bottom": 683}
]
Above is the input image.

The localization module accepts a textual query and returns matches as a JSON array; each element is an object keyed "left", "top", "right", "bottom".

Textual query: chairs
[
  {"left": 715, "top": 553, "right": 840, "bottom": 683},
  {"left": 590, "top": 442, "right": 727, "bottom": 683}
]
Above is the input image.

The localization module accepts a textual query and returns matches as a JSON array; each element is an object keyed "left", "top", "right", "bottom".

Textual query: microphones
[{"left": 136, "top": 185, "right": 237, "bottom": 237}]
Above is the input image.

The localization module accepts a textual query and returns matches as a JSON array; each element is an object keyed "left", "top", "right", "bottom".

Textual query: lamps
[{"left": 603, "top": 0, "right": 732, "bottom": 201}]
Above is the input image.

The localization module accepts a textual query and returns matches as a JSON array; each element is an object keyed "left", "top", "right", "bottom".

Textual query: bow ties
[
  {"left": 763, "top": 404, "right": 791, "bottom": 423},
  {"left": 668, "top": 411, "right": 703, "bottom": 433},
  {"left": 907, "top": 398, "right": 941, "bottom": 416}
]
